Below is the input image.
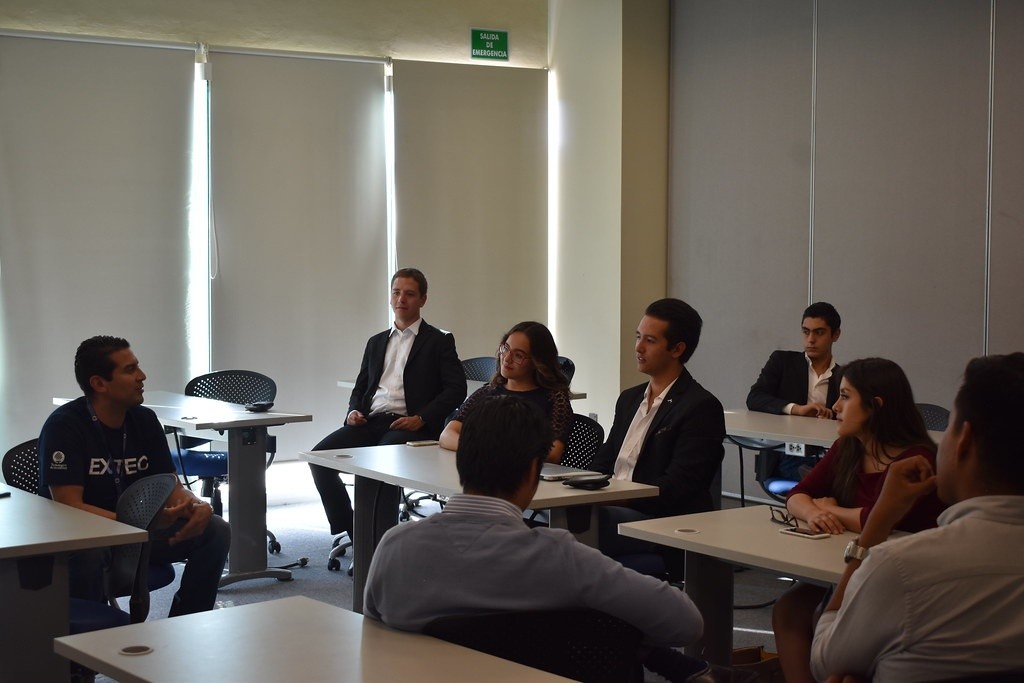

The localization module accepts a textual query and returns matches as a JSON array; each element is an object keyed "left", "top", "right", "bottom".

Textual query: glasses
[{"left": 499, "top": 342, "right": 531, "bottom": 364}]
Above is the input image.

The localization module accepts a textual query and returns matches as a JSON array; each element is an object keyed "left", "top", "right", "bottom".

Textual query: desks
[{"left": 0, "top": 391, "right": 953, "bottom": 683}]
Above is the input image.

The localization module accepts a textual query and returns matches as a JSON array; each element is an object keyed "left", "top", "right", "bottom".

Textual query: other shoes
[{"left": 668, "top": 655, "right": 713, "bottom": 683}]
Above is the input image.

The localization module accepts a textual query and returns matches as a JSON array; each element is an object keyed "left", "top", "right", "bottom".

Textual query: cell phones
[
  {"left": 406, "top": 440, "right": 439, "bottom": 447},
  {"left": 562, "top": 474, "right": 612, "bottom": 485},
  {"left": 779, "top": 527, "right": 831, "bottom": 539}
]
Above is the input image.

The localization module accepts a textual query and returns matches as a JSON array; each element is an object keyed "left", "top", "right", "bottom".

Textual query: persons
[
  {"left": 441, "top": 323, "right": 576, "bottom": 465},
  {"left": 361, "top": 395, "right": 710, "bottom": 683},
  {"left": 810, "top": 354, "right": 1024, "bottom": 683},
  {"left": 747, "top": 301, "right": 845, "bottom": 496},
  {"left": 772, "top": 357, "right": 948, "bottom": 683},
  {"left": 36, "top": 334, "right": 229, "bottom": 618},
  {"left": 565, "top": 299, "right": 724, "bottom": 555},
  {"left": 305, "top": 268, "right": 465, "bottom": 543}
]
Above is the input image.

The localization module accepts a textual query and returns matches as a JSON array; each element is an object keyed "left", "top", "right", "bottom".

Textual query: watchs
[
  {"left": 845, "top": 538, "right": 867, "bottom": 563},
  {"left": 198, "top": 501, "right": 214, "bottom": 518},
  {"left": 418, "top": 414, "right": 427, "bottom": 425}
]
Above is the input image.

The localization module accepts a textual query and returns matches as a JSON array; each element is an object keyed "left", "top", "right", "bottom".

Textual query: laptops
[{"left": 539, "top": 462, "right": 603, "bottom": 481}]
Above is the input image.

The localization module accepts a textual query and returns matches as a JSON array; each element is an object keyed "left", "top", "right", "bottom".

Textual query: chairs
[{"left": 1, "top": 348, "right": 954, "bottom": 614}]
